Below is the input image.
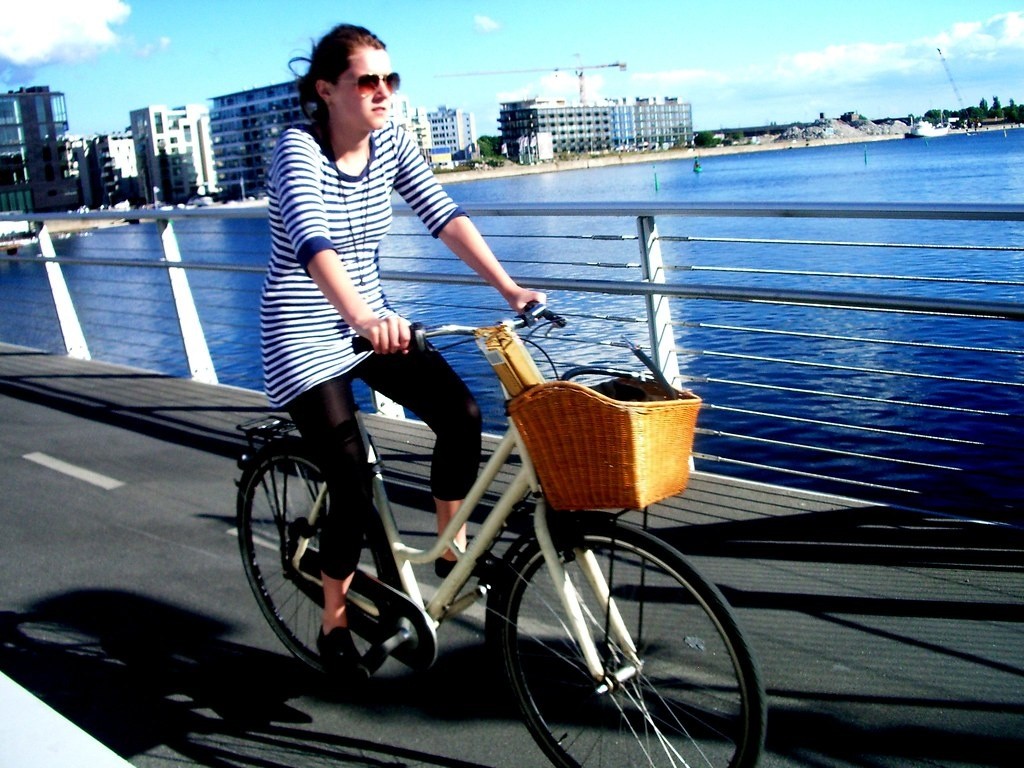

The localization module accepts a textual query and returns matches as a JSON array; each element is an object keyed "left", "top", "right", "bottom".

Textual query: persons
[{"left": 261, "top": 23, "right": 547, "bottom": 671}]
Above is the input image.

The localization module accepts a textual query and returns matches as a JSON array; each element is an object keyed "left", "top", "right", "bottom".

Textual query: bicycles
[{"left": 232, "top": 299, "right": 770, "bottom": 768}]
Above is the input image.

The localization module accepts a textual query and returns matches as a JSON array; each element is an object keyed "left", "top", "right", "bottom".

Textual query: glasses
[{"left": 331, "top": 72, "right": 401, "bottom": 96}]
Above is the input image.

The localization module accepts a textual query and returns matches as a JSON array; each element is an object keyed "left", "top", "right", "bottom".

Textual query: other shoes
[
  {"left": 317, "top": 623, "right": 371, "bottom": 691},
  {"left": 435, "top": 541, "right": 499, "bottom": 578}
]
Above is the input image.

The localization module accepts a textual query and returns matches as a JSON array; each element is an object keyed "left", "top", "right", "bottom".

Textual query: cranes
[
  {"left": 935, "top": 47, "right": 973, "bottom": 128},
  {"left": 433, "top": 51, "right": 628, "bottom": 103}
]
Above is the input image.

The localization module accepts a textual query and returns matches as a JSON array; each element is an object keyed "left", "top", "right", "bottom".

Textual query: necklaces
[{"left": 330, "top": 136, "right": 369, "bottom": 286}]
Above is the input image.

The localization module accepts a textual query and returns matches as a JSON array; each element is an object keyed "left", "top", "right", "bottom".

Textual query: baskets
[{"left": 506, "top": 363, "right": 703, "bottom": 512}]
